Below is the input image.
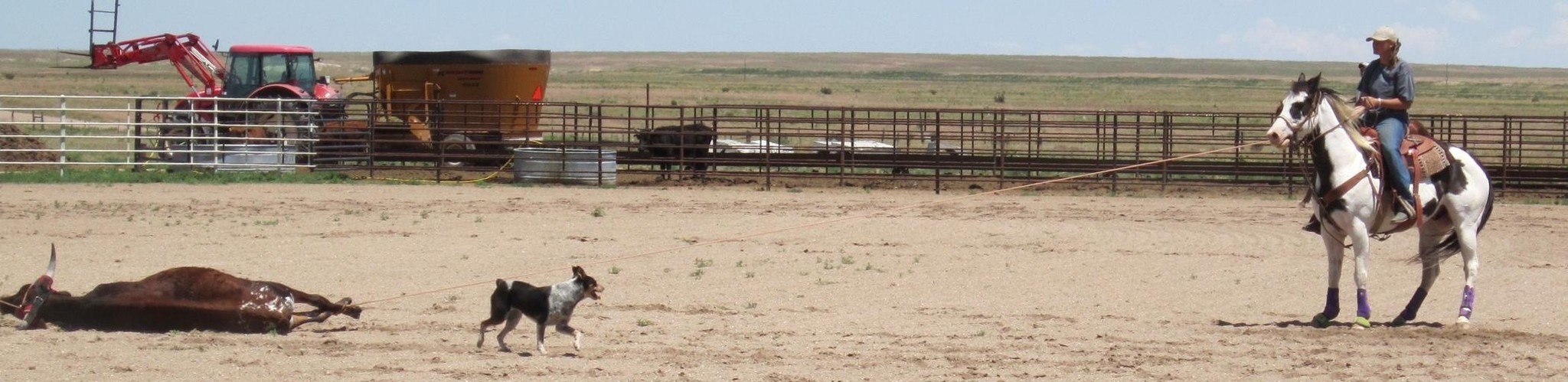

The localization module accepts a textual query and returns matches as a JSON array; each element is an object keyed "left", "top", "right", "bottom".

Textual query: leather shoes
[
  {"left": 1390, "top": 195, "right": 1416, "bottom": 224},
  {"left": 1301, "top": 219, "right": 1321, "bottom": 236}
]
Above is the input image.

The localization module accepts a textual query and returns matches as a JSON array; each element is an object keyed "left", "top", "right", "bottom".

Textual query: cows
[
  {"left": 631, "top": 123, "right": 714, "bottom": 181},
  {"left": 0, "top": 238, "right": 362, "bottom": 336}
]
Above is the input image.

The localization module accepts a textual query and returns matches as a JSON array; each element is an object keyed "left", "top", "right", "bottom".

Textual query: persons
[{"left": 1355, "top": 27, "right": 1416, "bottom": 223}]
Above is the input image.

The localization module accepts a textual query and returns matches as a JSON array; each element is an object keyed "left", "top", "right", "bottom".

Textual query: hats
[
  {"left": 1366, "top": 26, "right": 1398, "bottom": 43},
  {"left": 1358, "top": 61, "right": 1369, "bottom": 69}
]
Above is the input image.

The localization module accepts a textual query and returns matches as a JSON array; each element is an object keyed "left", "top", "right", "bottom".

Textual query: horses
[{"left": 1264, "top": 68, "right": 1496, "bottom": 331}]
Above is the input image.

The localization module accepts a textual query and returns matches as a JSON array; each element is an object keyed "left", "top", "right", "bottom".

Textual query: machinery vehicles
[{"left": 89, "top": 30, "right": 346, "bottom": 165}]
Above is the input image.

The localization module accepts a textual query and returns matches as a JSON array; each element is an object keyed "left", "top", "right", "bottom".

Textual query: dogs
[{"left": 475, "top": 264, "right": 605, "bottom": 356}]
